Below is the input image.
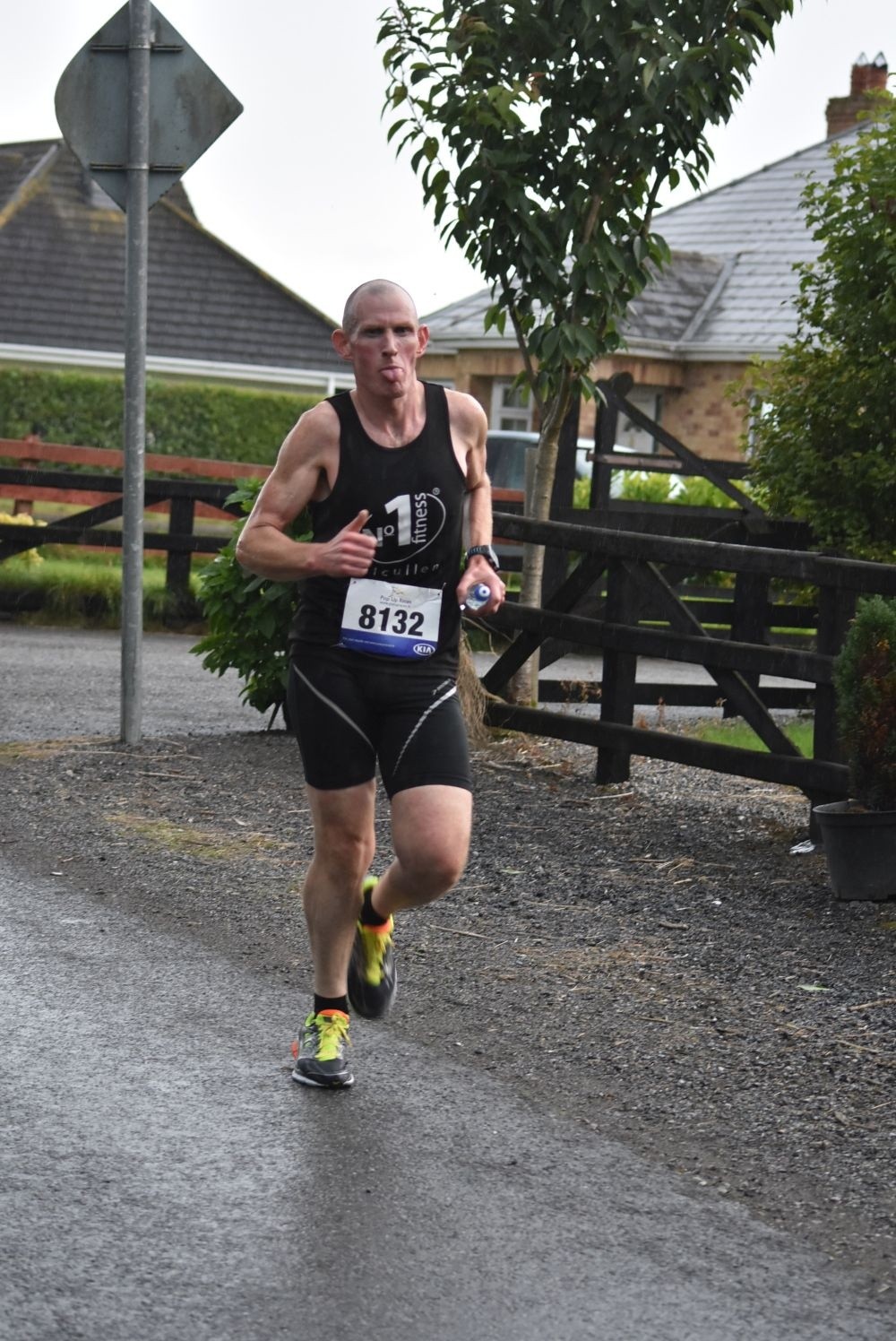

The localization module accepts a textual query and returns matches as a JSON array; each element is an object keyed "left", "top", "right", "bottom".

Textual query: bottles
[{"left": 464, "top": 582, "right": 491, "bottom": 611}]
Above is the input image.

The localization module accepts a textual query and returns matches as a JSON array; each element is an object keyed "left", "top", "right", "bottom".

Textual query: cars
[{"left": 486, "top": 428, "right": 691, "bottom": 502}]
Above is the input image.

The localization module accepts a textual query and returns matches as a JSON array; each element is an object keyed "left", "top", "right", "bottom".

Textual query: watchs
[{"left": 463, "top": 545, "right": 500, "bottom": 571}]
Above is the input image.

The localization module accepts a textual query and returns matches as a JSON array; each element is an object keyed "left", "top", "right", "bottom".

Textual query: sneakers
[
  {"left": 345, "top": 875, "right": 399, "bottom": 1023},
  {"left": 288, "top": 1008, "right": 358, "bottom": 1087}
]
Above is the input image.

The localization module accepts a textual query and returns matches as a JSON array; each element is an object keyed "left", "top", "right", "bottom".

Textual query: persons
[{"left": 236, "top": 278, "right": 506, "bottom": 1089}]
